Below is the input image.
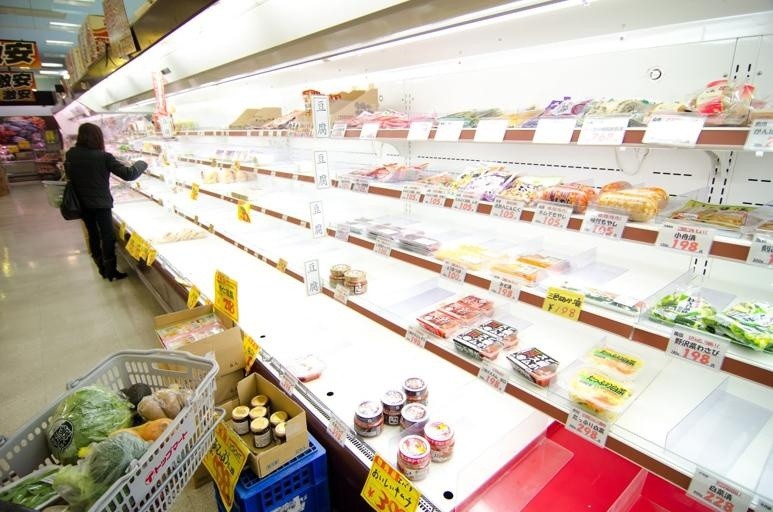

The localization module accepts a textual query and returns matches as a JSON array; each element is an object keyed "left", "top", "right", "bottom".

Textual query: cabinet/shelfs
[{"left": 0, "top": 105, "right": 61, "bottom": 183}]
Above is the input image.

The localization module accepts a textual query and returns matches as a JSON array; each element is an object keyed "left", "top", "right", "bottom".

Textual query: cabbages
[
  {"left": 87, "top": 431, "right": 147, "bottom": 480},
  {"left": 46, "top": 385, "right": 132, "bottom": 465}
]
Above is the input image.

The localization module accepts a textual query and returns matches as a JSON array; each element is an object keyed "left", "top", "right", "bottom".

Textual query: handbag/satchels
[{"left": 60, "top": 181, "right": 84, "bottom": 222}]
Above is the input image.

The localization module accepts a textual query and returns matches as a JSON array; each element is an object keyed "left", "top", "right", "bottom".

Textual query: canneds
[
  {"left": 250, "top": 394, "right": 269, "bottom": 407},
  {"left": 275, "top": 422, "right": 286, "bottom": 445},
  {"left": 402, "top": 378, "right": 428, "bottom": 405},
  {"left": 269, "top": 411, "right": 287, "bottom": 439},
  {"left": 231, "top": 406, "right": 251, "bottom": 435},
  {"left": 248, "top": 407, "right": 268, "bottom": 419},
  {"left": 250, "top": 418, "right": 270, "bottom": 448},
  {"left": 400, "top": 404, "right": 427, "bottom": 432},
  {"left": 380, "top": 390, "right": 405, "bottom": 426},
  {"left": 396, "top": 434, "right": 431, "bottom": 482},
  {"left": 424, "top": 421, "right": 453, "bottom": 462},
  {"left": 328, "top": 264, "right": 367, "bottom": 296},
  {"left": 354, "top": 401, "right": 384, "bottom": 436}
]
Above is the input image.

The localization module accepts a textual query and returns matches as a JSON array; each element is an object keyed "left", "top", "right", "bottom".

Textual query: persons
[{"left": 63, "top": 123, "right": 152, "bottom": 282}]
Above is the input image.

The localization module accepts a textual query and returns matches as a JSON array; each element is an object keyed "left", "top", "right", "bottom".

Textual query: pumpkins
[{"left": 105, "top": 416, "right": 172, "bottom": 442}]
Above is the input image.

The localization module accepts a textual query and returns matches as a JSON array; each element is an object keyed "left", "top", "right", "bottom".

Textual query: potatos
[{"left": 136, "top": 387, "right": 192, "bottom": 419}]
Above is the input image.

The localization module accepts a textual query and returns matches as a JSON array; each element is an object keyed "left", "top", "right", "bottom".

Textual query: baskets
[{"left": 1, "top": 350, "right": 332, "bottom": 512}]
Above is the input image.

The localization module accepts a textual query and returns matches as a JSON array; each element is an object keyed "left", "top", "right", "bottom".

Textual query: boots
[{"left": 92, "top": 254, "right": 129, "bottom": 283}]
